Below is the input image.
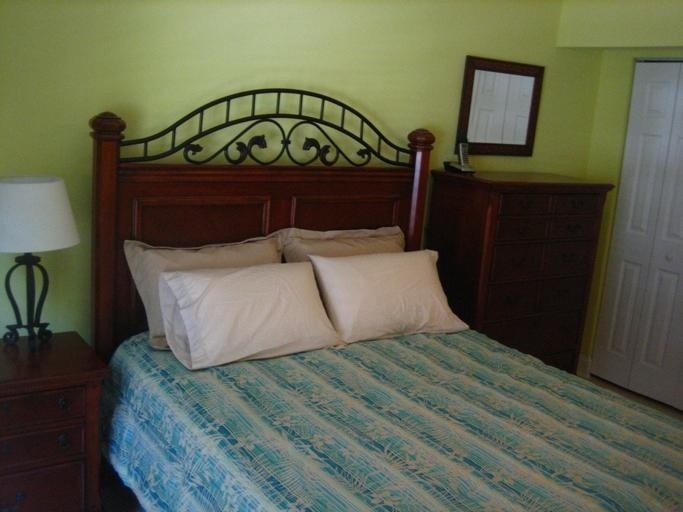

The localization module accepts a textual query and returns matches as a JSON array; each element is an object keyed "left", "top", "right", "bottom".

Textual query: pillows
[
  {"left": 122, "top": 229, "right": 286, "bottom": 355},
  {"left": 307, "top": 249, "right": 469, "bottom": 348},
  {"left": 280, "top": 227, "right": 410, "bottom": 269},
  {"left": 158, "top": 260, "right": 346, "bottom": 374}
]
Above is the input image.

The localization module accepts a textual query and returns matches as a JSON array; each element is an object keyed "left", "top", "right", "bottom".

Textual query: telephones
[{"left": 443, "top": 143, "right": 475, "bottom": 173}]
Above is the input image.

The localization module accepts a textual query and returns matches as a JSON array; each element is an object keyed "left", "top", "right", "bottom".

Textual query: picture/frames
[{"left": 454, "top": 55, "right": 545, "bottom": 156}]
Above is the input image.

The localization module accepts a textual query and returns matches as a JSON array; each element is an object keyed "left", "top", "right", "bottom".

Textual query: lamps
[{"left": 0, "top": 177, "right": 81, "bottom": 354}]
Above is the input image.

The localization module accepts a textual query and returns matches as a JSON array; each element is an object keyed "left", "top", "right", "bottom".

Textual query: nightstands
[
  {"left": 0, "top": 331, "right": 110, "bottom": 512},
  {"left": 424, "top": 170, "right": 615, "bottom": 374}
]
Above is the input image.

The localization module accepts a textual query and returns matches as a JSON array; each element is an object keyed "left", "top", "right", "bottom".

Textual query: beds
[{"left": 89, "top": 88, "right": 682, "bottom": 512}]
